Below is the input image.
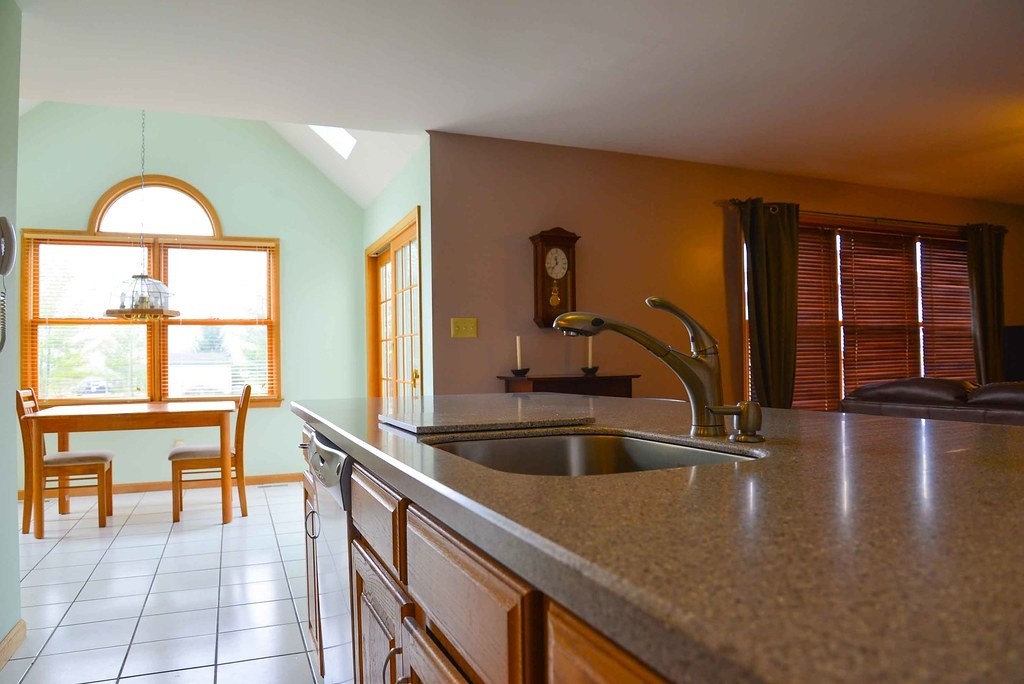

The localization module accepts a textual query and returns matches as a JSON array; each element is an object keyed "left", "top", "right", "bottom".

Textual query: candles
[
  {"left": 588, "top": 336, "right": 593, "bottom": 368},
  {"left": 516, "top": 336, "right": 521, "bottom": 370}
]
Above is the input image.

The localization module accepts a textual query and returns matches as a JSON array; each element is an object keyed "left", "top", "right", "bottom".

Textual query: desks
[
  {"left": 496, "top": 374, "right": 642, "bottom": 398},
  {"left": 20, "top": 401, "right": 236, "bottom": 539}
]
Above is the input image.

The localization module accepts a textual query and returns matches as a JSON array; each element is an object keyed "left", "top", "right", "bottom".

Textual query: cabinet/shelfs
[
  {"left": 542, "top": 596, "right": 666, "bottom": 684},
  {"left": 349, "top": 463, "right": 541, "bottom": 684},
  {"left": 299, "top": 424, "right": 324, "bottom": 677}
]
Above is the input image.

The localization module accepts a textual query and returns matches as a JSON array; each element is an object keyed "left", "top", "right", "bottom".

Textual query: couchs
[{"left": 840, "top": 376, "right": 1024, "bottom": 427}]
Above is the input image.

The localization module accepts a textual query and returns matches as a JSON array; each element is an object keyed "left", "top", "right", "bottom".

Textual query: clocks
[{"left": 529, "top": 227, "right": 581, "bottom": 329}]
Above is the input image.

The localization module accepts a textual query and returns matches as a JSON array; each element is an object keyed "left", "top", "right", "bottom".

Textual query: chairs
[
  {"left": 16, "top": 387, "right": 113, "bottom": 535},
  {"left": 169, "top": 384, "right": 251, "bottom": 523}
]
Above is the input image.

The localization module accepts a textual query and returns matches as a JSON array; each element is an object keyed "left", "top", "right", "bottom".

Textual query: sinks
[{"left": 421, "top": 425, "right": 766, "bottom": 481}]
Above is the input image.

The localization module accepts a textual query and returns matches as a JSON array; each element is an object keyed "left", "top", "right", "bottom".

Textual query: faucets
[
  {"left": 550, "top": 293, "right": 727, "bottom": 439},
  {"left": 702, "top": 400, "right": 766, "bottom": 443}
]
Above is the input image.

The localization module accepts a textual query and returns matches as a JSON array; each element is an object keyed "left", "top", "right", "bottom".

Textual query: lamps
[{"left": 106, "top": 111, "right": 180, "bottom": 321}]
